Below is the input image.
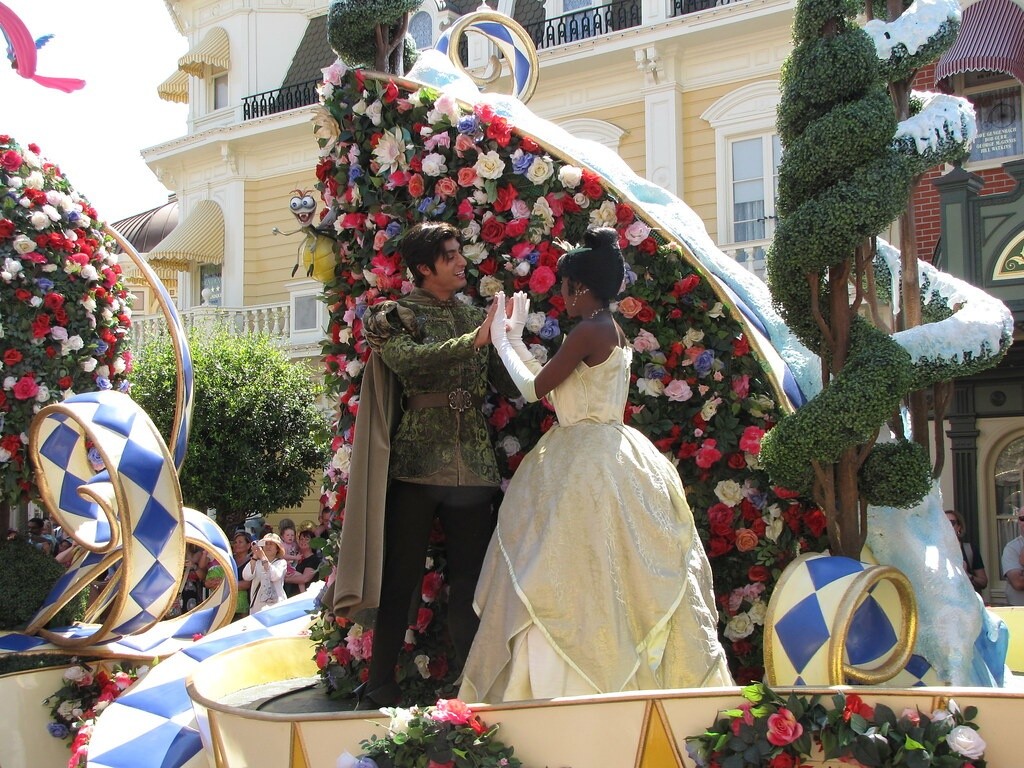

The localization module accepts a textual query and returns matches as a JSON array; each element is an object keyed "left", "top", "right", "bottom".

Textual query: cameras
[{"left": 251, "top": 540, "right": 266, "bottom": 548}]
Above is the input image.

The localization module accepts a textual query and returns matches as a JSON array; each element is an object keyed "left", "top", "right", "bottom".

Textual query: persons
[
  {"left": 1000, "top": 506, "right": 1024, "bottom": 609},
  {"left": 451, "top": 227, "right": 736, "bottom": 710},
  {"left": 6, "top": 504, "right": 329, "bottom": 627},
  {"left": 324, "top": 221, "right": 513, "bottom": 709},
  {"left": 945, "top": 509, "right": 989, "bottom": 597}
]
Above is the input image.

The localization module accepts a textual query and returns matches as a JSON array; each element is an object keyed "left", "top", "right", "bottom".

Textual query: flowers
[
  {"left": 298, "top": 58, "right": 991, "bottom": 768},
  {"left": 0, "top": 132, "right": 133, "bottom": 515},
  {"left": 39, "top": 653, "right": 162, "bottom": 768}
]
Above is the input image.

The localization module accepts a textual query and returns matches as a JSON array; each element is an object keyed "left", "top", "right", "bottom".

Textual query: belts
[{"left": 406, "top": 390, "right": 483, "bottom": 409}]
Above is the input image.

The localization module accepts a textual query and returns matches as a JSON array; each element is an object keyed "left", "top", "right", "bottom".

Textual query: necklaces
[{"left": 590, "top": 308, "right": 612, "bottom": 320}]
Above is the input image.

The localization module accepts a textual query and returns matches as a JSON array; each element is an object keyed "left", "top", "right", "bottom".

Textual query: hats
[{"left": 257, "top": 532, "right": 285, "bottom": 558}]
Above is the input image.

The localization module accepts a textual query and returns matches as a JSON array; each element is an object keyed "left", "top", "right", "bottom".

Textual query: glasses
[
  {"left": 950, "top": 520, "right": 960, "bottom": 527},
  {"left": 1019, "top": 516, "right": 1024, "bottom": 521}
]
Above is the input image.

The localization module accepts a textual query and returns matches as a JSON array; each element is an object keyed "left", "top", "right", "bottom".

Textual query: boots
[{"left": 353, "top": 677, "right": 403, "bottom": 711}]
